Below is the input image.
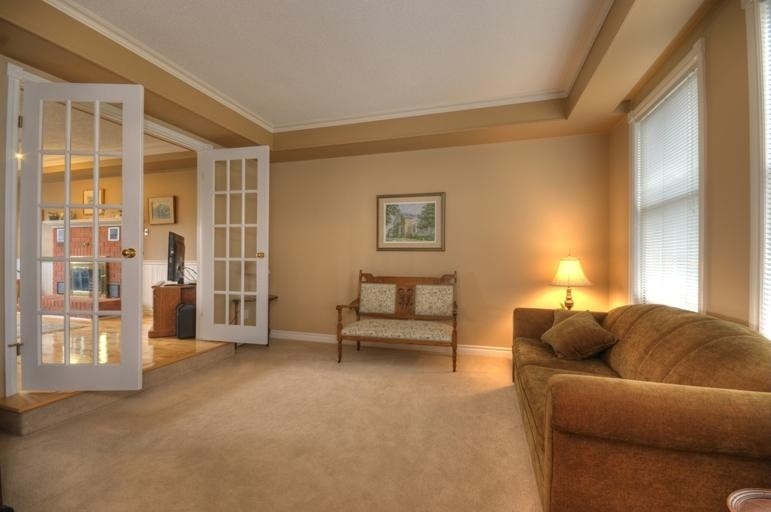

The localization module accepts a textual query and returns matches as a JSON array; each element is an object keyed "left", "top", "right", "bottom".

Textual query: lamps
[{"left": 549, "top": 254, "right": 594, "bottom": 310}]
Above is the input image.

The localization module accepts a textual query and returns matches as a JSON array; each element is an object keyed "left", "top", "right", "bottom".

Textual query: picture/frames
[
  {"left": 148, "top": 196, "right": 175, "bottom": 225},
  {"left": 83, "top": 189, "right": 103, "bottom": 215},
  {"left": 56, "top": 229, "right": 64, "bottom": 242},
  {"left": 376, "top": 192, "right": 445, "bottom": 252},
  {"left": 108, "top": 227, "right": 120, "bottom": 241}
]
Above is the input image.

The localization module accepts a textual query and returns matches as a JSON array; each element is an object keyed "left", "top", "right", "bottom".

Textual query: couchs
[
  {"left": 512, "top": 301, "right": 770, "bottom": 511},
  {"left": 336, "top": 270, "right": 457, "bottom": 372}
]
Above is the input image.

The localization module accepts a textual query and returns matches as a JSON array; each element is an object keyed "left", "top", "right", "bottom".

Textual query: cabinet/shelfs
[{"left": 146, "top": 280, "right": 196, "bottom": 338}]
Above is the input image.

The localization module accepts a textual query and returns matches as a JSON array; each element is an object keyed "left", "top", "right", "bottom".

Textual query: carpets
[{"left": 16, "top": 317, "right": 89, "bottom": 339}]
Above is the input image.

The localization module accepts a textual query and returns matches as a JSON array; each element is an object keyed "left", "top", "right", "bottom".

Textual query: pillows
[
  {"left": 552, "top": 310, "right": 579, "bottom": 327},
  {"left": 541, "top": 310, "right": 619, "bottom": 360}
]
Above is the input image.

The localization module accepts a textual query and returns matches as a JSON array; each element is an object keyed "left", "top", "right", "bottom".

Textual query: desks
[{"left": 232, "top": 293, "right": 278, "bottom": 349}]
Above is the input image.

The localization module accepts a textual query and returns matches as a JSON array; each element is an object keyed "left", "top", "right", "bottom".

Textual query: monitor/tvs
[{"left": 164, "top": 231, "right": 185, "bottom": 286}]
